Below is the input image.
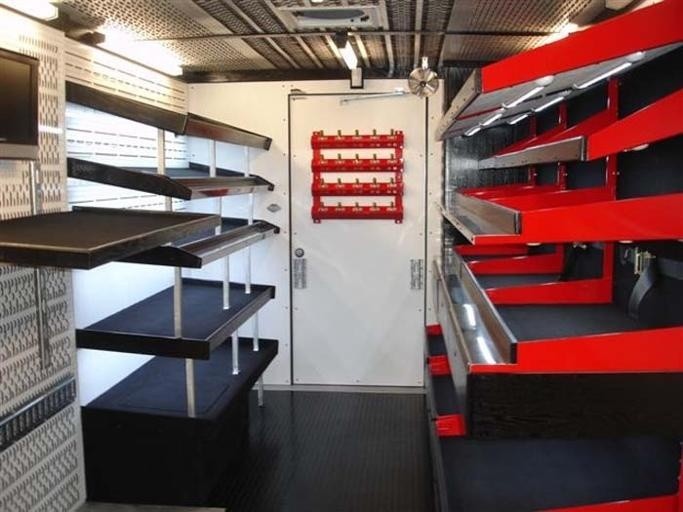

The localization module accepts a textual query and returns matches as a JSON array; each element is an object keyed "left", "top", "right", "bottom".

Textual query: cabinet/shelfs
[
  {"left": 64, "top": 81, "right": 280, "bottom": 489},
  {"left": 420, "top": 0, "right": 682, "bottom": 512}
]
